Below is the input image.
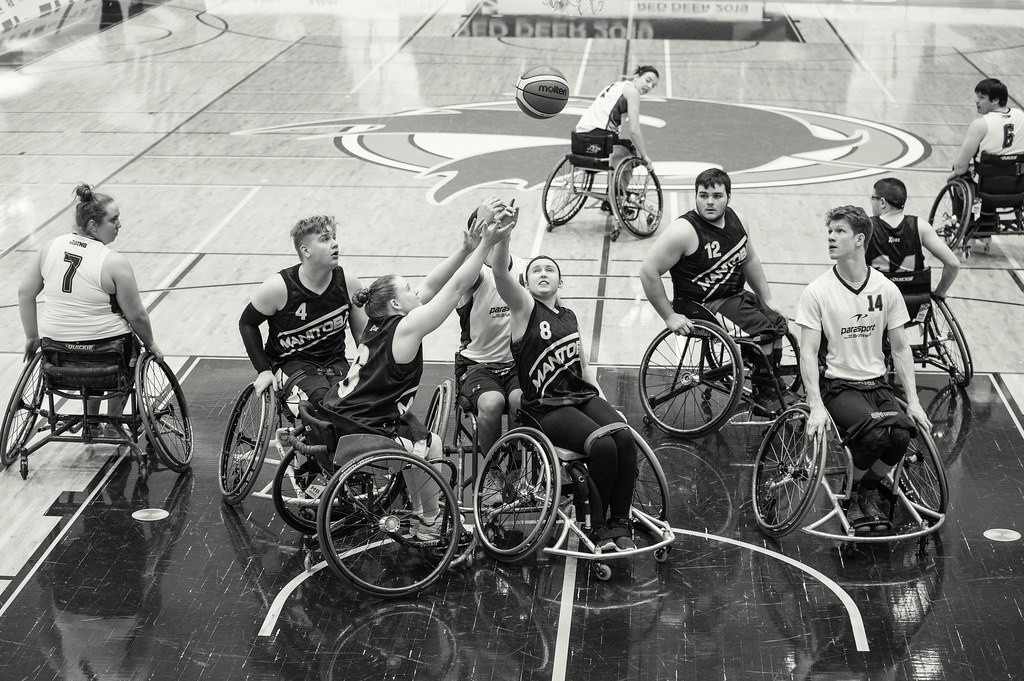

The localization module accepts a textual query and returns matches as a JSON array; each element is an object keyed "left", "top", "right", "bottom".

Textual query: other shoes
[
  {"left": 103, "top": 424, "right": 131, "bottom": 439},
  {"left": 85, "top": 423, "right": 102, "bottom": 437}
]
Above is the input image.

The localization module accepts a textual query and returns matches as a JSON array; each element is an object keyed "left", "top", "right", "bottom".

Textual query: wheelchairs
[
  {"left": 472, "top": 408, "right": 675, "bottom": 581},
  {"left": 927, "top": 150, "right": 1024, "bottom": 261},
  {"left": 218, "top": 379, "right": 538, "bottom": 603},
  {"left": 638, "top": 284, "right": 802, "bottom": 441},
  {"left": 752, "top": 396, "right": 950, "bottom": 562},
  {"left": 878, "top": 266, "right": 974, "bottom": 398},
  {"left": 541, "top": 131, "right": 663, "bottom": 243},
  {"left": 0, "top": 336, "right": 195, "bottom": 486}
]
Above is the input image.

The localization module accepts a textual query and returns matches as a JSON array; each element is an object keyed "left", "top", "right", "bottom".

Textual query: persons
[
  {"left": 18, "top": 183, "right": 163, "bottom": 439},
  {"left": 576, "top": 65, "right": 659, "bottom": 219},
  {"left": 328, "top": 218, "right": 516, "bottom": 540},
  {"left": 638, "top": 168, "right": 795, "bottom": 413},
  {"left": 493, "top": 198, "right": 638, "bottom": 553},
  {"left": 460, "top": 198, "right": 534, "bottom": 507},
  {"left": 239, "top": 217, "right": 368, "bottom": 410},
  {"left": 948, "top": 79, "right": 1024, "bottom": 235},
  {"left": 866, "top": 176, "right": 959, "bottom": 314},
  {"left": 795, "top": 205, "right": 932, "bottom": 534}
]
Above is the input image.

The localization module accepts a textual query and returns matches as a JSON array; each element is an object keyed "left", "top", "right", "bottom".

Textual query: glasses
[{"left": 871, "top": 196, "right": 880, "bottom": 201}]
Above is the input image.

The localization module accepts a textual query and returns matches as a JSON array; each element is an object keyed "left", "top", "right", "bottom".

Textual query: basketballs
[{"left": 514, "top": 63, "right": 570, "bottom": 120}]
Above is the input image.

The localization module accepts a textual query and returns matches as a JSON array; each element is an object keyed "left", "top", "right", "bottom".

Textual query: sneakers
[
  {"left": 483, "top": 470, "right": 507, "bottom": 507},
  {"left": 417, "top": 511, "right": 454, "bottom": 540},
  {"left": 410, "top": 511, "right": 465, "bottom": 538},
  {"left": 605, "top": 518, "right": 637, "bottom": 551},
  {"left": 754, "top": 388, "right": 796, "bottom": 416},
  {"left": 859, "top": 490, "right": 890, "bottom": 532},
  {"left": 841, "top": 490, "right": 870, "bottom": 536},
  {"left": 503, "top": 464, "right": 527, "bottom": 499},
  {"left": 588, "top": 526, "right": 615, "bottom": 552}
]
